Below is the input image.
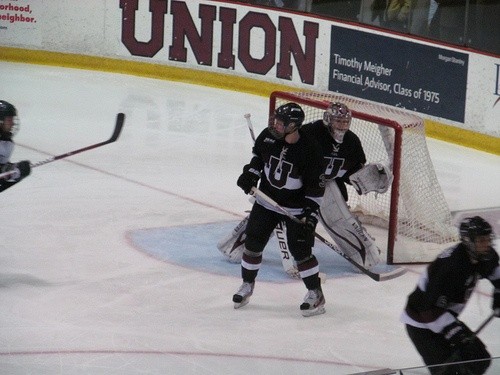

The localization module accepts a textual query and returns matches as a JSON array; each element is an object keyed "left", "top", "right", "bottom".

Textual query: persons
[
  {"left": 238, "top": 0, "right": 500, "bottom": 56},
  {"left": 0, "top": 101, "right": 30, "bottom": 193},
  {"left": 402, "top": 216, "right": 500, "bottom": 375},
  {"left": 232, "top": 103, "right": 326, "bottom": 317},
  {"left": 216, "top": 101, "right": 394, "bottom": 274}
]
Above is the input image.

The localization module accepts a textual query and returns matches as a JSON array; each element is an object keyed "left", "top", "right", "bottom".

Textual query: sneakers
[
  {"left": 232, "top": 280, "right": 255, "bottom": 308},
  {"left": 300, "top": 285, "right": 326, "bottom": 316}
]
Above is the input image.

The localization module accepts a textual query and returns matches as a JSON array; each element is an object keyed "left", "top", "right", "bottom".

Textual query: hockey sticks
[
  {"left": 245, "top": 113, "right": 326, "bottom": 281},
  {"left": 474, "top": 312, "right": 497, "bottom": 335},
  {"left": 1, "top": 113, "right": 126, "bottom": 177},
  {"left": 252, "top": 187, "right": 407, "bottom": 281}
]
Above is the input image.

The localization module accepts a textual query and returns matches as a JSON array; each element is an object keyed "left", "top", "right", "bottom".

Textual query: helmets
[
  {"left": 322, "top": 103, "right": 353, "bottom": 127},
  {"left": 272, "top": 102, "right": 305, "bottom": 128},
  {"left": 459, "top": 215, "right": 493, "bottom": 241}
]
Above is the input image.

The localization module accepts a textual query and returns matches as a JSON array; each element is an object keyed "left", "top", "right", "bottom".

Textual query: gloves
[
  {"left": 441, "top": 321, "right": 476, "bottom": 358},
  {"left": 297, "top": 202, "right": 319, "bottom": 245},
  {"left": 236, "top": 164, "right": 262, "bottom": 194},
  {"left": 490, "top": 290, "right": 500, "bottom": 318}
]
